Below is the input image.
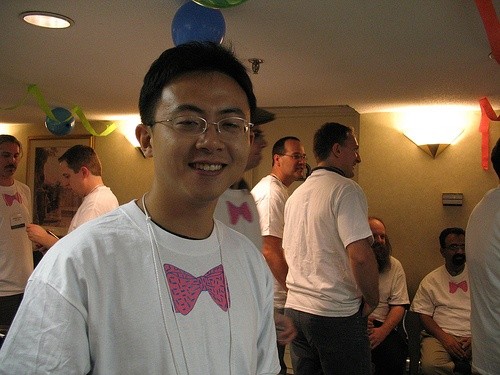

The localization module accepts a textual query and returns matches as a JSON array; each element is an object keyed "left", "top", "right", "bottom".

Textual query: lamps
[{"left": 401, "top": 118, "right": 463, "bottom": 159}]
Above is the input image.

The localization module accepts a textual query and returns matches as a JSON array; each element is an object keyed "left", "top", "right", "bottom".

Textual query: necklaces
[{"left": 142, "top": 192, "right": 232, "bottom": 375}]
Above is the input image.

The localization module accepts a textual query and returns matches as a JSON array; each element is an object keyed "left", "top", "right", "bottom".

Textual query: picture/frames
[{"left": 25, "top": 133, "right": 94, "bottom": 239}]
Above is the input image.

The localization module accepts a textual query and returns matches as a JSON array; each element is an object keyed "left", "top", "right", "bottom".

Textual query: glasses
[
  {"left": 149, "top": 115, "right": 254, "bottom": 138},
  {"left": 279, "top": 153, "right": 306, "bottom": 159}
]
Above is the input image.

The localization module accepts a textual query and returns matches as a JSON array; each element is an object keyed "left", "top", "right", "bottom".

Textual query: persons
[
  {"left": 465, "top": 138, "right": 500, "bottom": 375},
  {"left": 0, "top": 40, "right": 281, "bottom": 375},
  {"left": 367, "top": 217, "right": 411, "bottom": 375},
  {"left": 250, "top": 122, "right": 380, "bottom": 375},
  {"left": 410, "top": 227, "right": 472, "bottom": 375}
]
